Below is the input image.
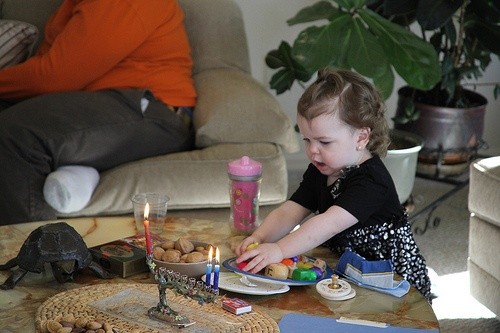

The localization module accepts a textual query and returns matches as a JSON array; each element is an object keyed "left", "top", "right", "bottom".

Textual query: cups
[{"left": 130, "top": 193, "right": 170, "bottom": 236}]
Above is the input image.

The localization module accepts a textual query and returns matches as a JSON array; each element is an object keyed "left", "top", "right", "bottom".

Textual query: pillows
[{"left": 0, "top": 18, "right": 38, "bottom": 69}]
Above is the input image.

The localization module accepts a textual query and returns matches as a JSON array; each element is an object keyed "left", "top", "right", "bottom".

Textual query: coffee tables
[{"left": 0, "top": 218, "right": 440, "bottom": 333}]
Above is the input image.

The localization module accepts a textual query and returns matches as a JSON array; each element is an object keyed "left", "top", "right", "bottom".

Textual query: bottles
[{"left": 227, "top": 156, "right": 262, "bottom": 235}]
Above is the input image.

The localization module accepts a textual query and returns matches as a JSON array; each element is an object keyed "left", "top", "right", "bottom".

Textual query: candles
[
  {"left": 143, "top": 202, "right": 152, "bottom": 253},
  {"left": 213, "top": 247, "right": 220, "bottom": 289},
  {"left": 206, "top": 246, "right": 212, "bottom": 286}
]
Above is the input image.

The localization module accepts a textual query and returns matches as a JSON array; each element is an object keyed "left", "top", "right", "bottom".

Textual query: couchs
[{"left": 0, "top": 0, "right": 300, "bottom": 217}]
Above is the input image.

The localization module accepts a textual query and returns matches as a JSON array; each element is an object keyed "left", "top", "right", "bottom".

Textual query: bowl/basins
[{"left": 150, "top": 239, "right": 216, "bottom": 277}]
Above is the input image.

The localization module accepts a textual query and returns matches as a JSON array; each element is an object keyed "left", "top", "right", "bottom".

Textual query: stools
[{"left": 468, "top": 156, "right": 500, "bottom": 316}]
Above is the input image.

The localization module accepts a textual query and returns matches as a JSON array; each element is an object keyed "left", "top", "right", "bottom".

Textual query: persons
[
  {"left": 235, "top": 69, "right": 432, "bottom": 306},
  {"left": 0, "top": 0, "right": 198, "bottom": 223}
]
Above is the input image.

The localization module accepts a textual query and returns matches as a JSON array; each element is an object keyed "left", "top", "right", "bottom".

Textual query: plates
[
  {"left": 201, "top": 272, "right": 290, "bottom": 295},
  {"left": 221, "top": 257, "right": 333, "bottom": 285}
]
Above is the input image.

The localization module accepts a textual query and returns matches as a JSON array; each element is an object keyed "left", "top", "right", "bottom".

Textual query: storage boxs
[{"left": 221, "top": 297, "right": 251, "bottom": 314}]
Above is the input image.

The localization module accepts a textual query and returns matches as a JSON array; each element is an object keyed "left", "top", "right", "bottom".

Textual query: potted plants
[
  {"left": 391, "top": 0, "right": 500, "bottom": 164},
  {"left": 265, "top": 0, "right": 424, "bottom": 205}
]
